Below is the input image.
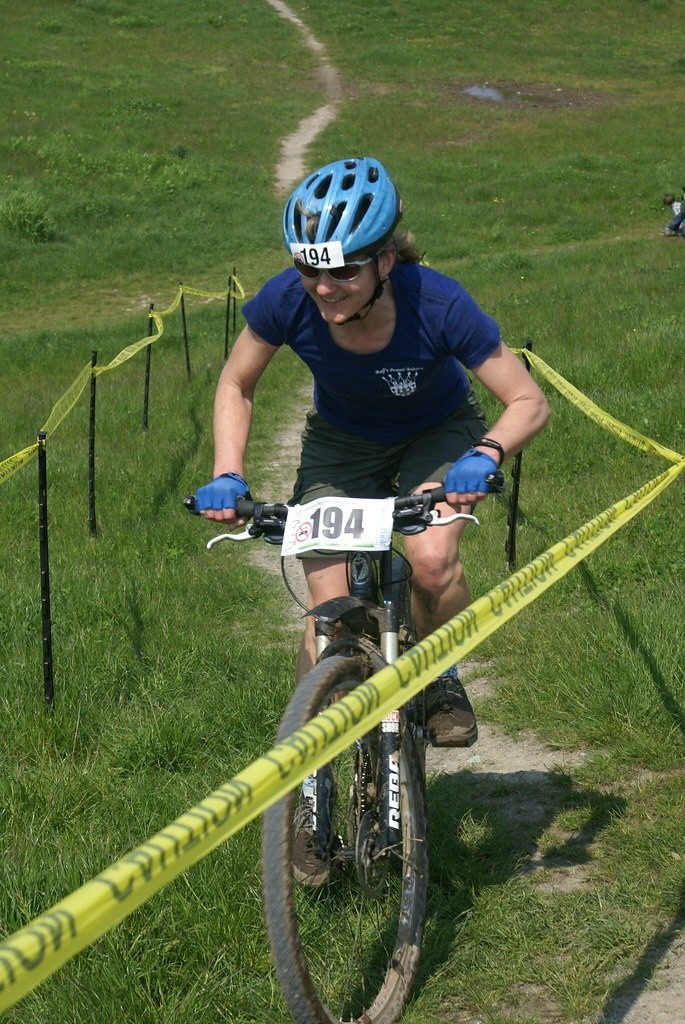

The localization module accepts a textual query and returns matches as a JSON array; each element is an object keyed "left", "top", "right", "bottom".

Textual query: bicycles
[{"left": 181, "top": 493, "right": 481, "bottom": 1022}]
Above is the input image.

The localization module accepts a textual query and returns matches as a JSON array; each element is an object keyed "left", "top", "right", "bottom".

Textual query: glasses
[{"left": 293, "top": 238, "right": 387, "bottom": 282}]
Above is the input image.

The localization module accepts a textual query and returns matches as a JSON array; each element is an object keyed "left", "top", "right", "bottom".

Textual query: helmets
[{"left": 282, "top": 156, "right": 405, "bottom": 268}]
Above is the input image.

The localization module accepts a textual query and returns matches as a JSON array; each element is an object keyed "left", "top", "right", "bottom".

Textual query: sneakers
[
  {"left": 290, "top": 789, "right": 336, "bottom": 886},
  {"left": 426, "top": 676, "right": 478, "bottom": 744}
]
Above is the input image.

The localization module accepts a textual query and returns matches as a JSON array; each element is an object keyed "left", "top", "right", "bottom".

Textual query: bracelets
[{"left": 473, "top": 437, "right": 504, "bottom": 469}]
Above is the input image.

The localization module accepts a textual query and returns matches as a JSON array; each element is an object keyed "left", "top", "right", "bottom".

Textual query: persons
[
  {"left": 194, "top": 156, "right": 552, "bottom": 888},
  {"left": 663, "top": 186, "right": 685, "bottom": 236}
]
